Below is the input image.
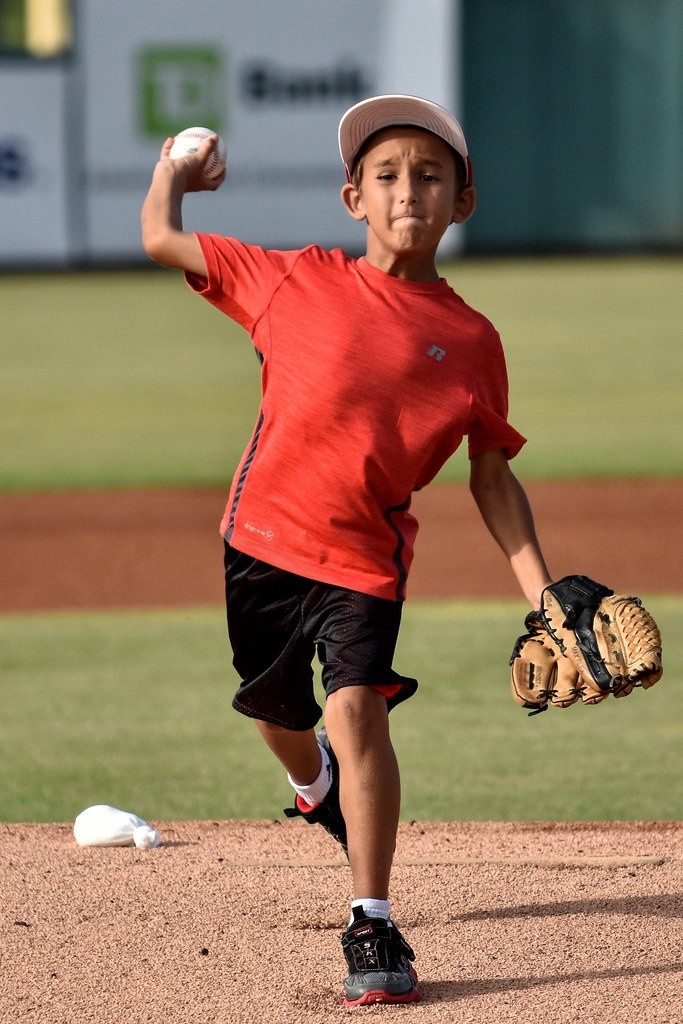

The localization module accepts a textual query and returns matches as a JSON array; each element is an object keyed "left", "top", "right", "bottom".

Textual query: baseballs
[{"left": 170, "top": 125, "right": 226, "bottom": 181}]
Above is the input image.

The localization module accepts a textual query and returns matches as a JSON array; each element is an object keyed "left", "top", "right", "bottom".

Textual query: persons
[{"left": 143, "top": 96, "right": 662, "bottom": 1005}]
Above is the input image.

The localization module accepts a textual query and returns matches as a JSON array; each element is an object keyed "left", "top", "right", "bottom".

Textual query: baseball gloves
[{"left": 510, "top": 571, "right": 661, "bottom": 718}]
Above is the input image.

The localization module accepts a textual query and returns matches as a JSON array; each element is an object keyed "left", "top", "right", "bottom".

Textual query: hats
[{"left": 338, "top": 93, "right": 472, "bottom": 189}]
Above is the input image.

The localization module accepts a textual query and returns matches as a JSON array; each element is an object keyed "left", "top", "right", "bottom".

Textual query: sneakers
[
  {"left": 340, "top": 904, "right": 422, "bottom": 1007},
  {"left": 282, "top": 725, "right": 349, "bottom": 864}
]
